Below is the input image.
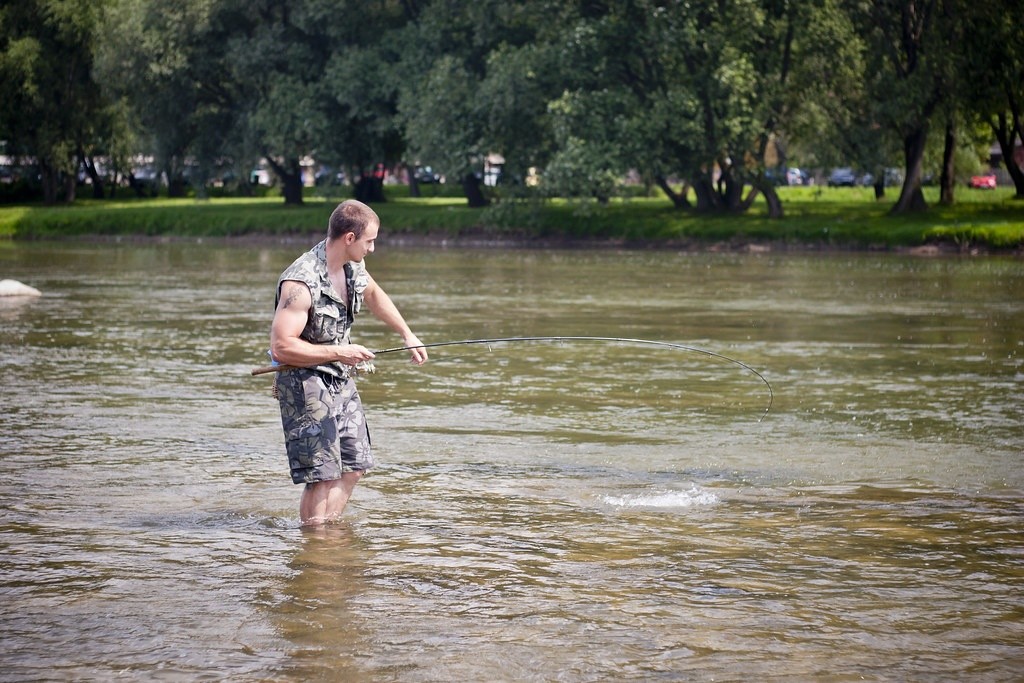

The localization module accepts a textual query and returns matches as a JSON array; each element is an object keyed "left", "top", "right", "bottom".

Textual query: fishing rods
[{"left": 250, "top": 336, "right": 777, "bottom": 424}]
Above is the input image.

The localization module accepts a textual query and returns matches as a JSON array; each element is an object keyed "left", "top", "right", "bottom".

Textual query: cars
[
  {"left": 414, "top": 166, "right": 442, "bottom": 185},
  {"left": 764, "top": 166, "right": 940, "bottom": 188},
  {"left": 968, "top": 169, "right": 996, "bottom": 190}
]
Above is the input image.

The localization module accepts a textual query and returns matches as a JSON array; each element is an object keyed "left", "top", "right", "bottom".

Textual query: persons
[{"left": 270, "top": 200, "right": 428, "bottom": 528}]
